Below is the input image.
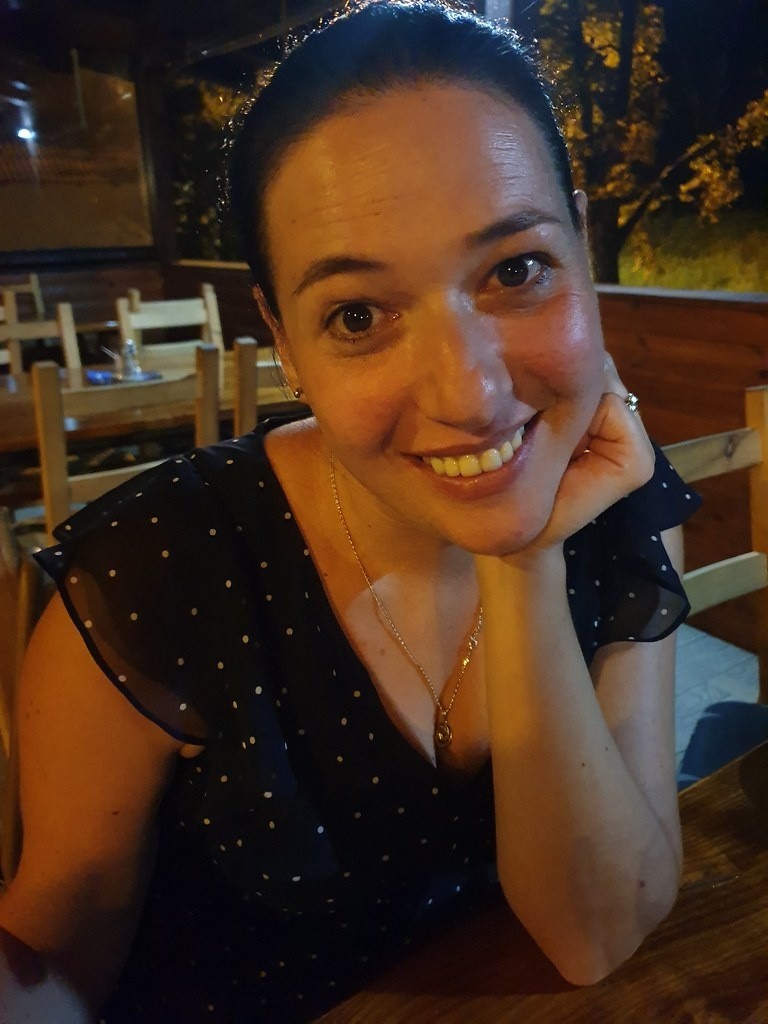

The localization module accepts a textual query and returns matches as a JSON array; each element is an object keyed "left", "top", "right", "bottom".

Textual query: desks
[
  {"left": 306, "top": 737, "right": 768, "bottom": 1024},
  {"left": 0, "top": 343, "right": 311, "bottom": 510}
]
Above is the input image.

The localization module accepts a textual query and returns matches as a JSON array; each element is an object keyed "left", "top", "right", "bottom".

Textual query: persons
[{"left": 1, "top": 0, "right": 685, "bottom": 1023}]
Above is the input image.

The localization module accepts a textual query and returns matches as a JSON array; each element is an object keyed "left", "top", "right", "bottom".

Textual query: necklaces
[{"left": 329, "top": 450, "right": 482, "bottom": 750}]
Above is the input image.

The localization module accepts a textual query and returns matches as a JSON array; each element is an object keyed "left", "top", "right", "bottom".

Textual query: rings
[{"left": 625, "top": 392, "right": 639, "bottom": 412}]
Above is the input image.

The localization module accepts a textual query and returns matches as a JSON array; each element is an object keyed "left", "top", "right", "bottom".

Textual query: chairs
[
  {"left": 659, "top": 384, "right": 767, "bottom": 785},
  {"left": 0, "top": 272, "right": 259, "bottom": 565}
]
